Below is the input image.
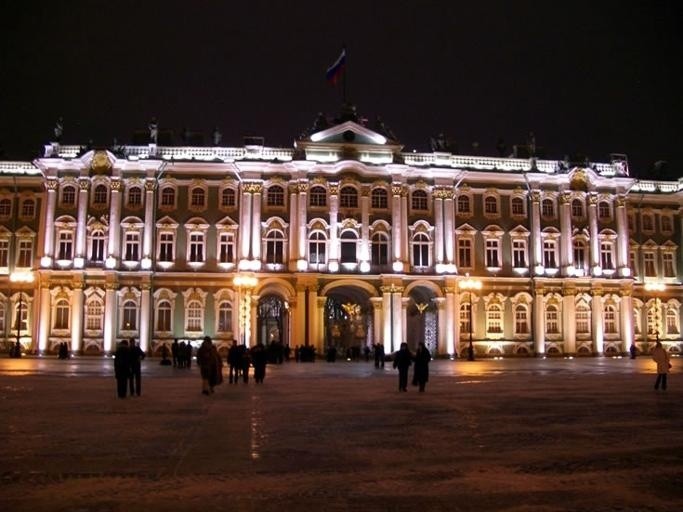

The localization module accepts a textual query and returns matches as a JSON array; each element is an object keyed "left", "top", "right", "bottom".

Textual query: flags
[{"left": 325, "top": 47, "right": 349, "bottom": 87}]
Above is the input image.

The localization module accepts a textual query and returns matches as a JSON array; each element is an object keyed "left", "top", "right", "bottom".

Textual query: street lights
[
  {"left": 457, "top": 278, "right": 483, "bottom": 362},
  {"left": 231, "top": 276, "right": 258, "bottom": 348},
  {"left": 9, "top": 271, "right": 35, "bottom": 357},
  {"left": 645, "top": 282, "right": 667, "bottom": 350}
]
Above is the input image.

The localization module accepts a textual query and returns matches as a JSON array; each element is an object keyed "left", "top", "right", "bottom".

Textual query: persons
[
  {"left": 630, "top": 344, "right": 636, "bottom": 359},
  {"left": 652, "top": 342, "right": 670, "bottom": 390},
  {"left": 226, "top": 340, "right": 267, "bottom": 385},
  {"left": 526, "top": 130, "right": 536, "bottom": 157},
  {"left": 171, "top": 339, "right": 193, "bottom": 368},
  {"left": 325, "top": 343, "right": 386, "bottom": 369},
  {"left": 8, "top": 342, "right": 21, "bottom": 358},
  {"left": 196, "top": 336, "right": 223, "bottom": 396},
  {"left": 114, "top": 338, "right": 145, "bottom": 397},
  {"left": 55, "top": 116, "right": 63, "bottom": 141},
  {"left": 268, "top": 340, "right": 291, "bottom": 366},
  {"left": 148, "top": 115, "right": 159, "bottom": 144},
  {"left": 430, "top": 128, "right": 450, "bottom": 152},
  {"left": 58, "top": 342, "right": 69, "bottom": 360},
  {"left": 210, "top": 127, "right": 222, "bottom": 147},
  {"left": 393, "top": 341, "right": 431, "bottom": 392},
  {"left": 295, "top": 344, "right": 316, "bottom": 362},
  {"left": 311, "top": 100, "right": 395, "bottom": 141}
]
